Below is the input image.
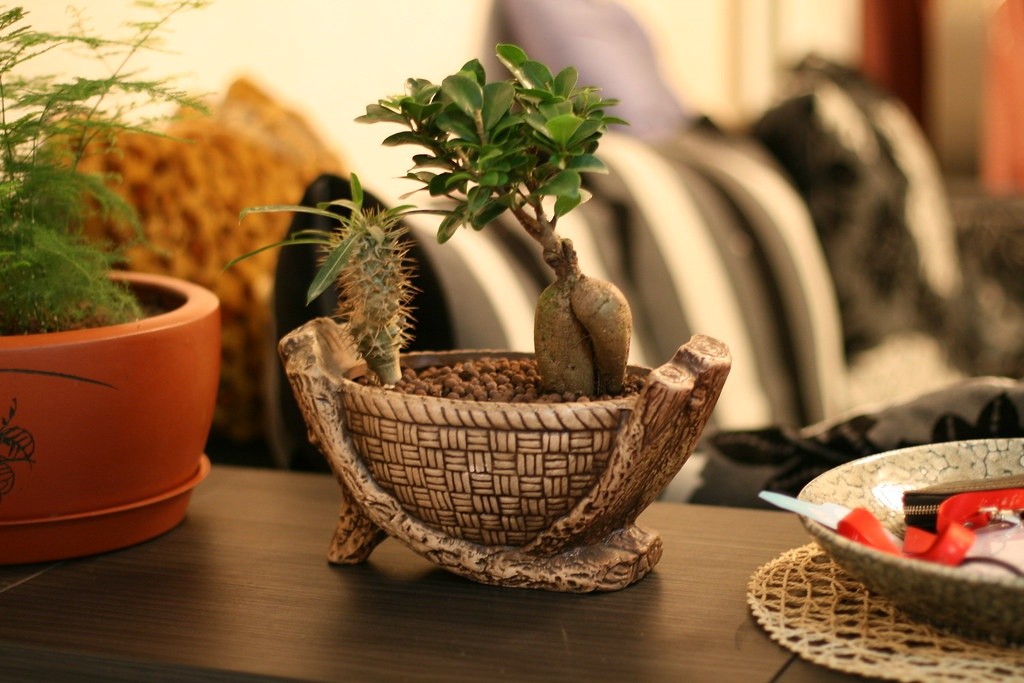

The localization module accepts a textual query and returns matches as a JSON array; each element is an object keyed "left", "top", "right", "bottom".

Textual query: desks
[{"left": 0, "top": 462, "right": 860, "bottom": 683}]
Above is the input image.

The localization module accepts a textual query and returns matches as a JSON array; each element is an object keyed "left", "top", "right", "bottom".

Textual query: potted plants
[
  {"left": 225, "top": 42, "right": 734, "bottom": 599},
  {"left": 0, "top": 0, "right": 229, "bottom": 567}
]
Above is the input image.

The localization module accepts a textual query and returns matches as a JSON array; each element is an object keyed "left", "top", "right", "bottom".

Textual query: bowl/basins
[{"left": 797, "top": 438, "right": 1024, "bottom": 644}]
